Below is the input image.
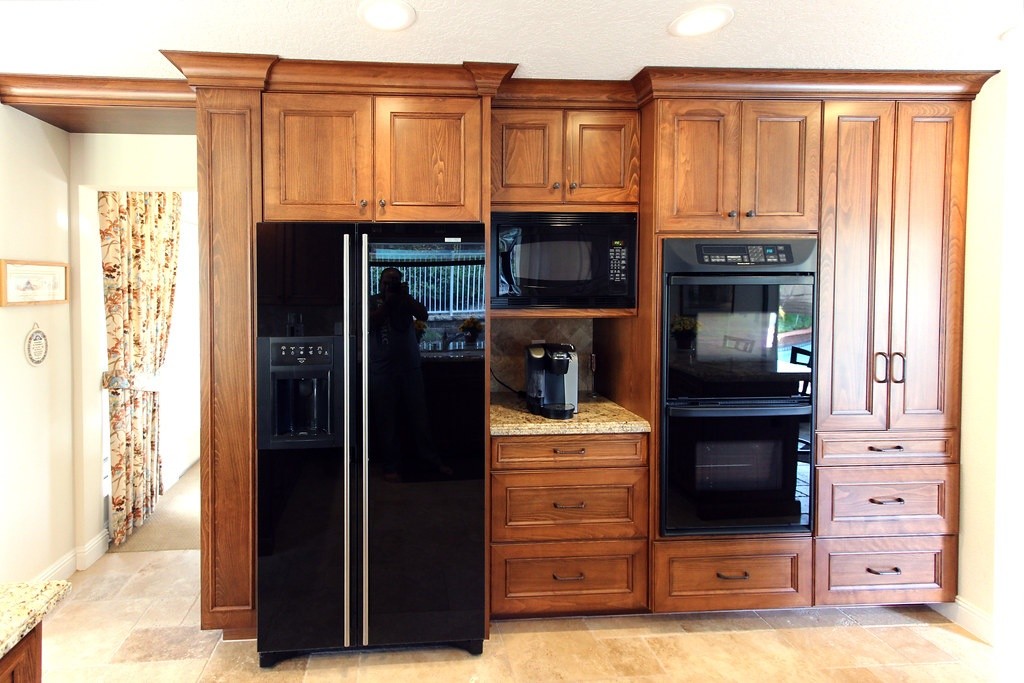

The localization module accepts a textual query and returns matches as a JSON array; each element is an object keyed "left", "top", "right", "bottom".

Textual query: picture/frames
[{"left": 0, "top": 258, "right": 70, "bottom": 307}]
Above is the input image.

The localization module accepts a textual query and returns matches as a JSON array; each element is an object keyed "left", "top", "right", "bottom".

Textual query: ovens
[{"left": 659, "top": 236, "right": 818, "bottom": 536}]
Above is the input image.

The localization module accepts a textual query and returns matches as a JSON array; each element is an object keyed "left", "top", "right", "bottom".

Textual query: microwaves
[{"left": 490, "top": 210, "right": 637, "bottom": 308}]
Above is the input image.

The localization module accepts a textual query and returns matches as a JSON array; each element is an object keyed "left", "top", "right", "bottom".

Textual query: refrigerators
[{"left": 249, "top": 222, "right": 486, "bottom": 666}]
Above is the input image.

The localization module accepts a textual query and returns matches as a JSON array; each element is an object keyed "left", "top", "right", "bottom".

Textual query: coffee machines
[{"left": 523, "top": 343, "right": 579, "bottom": 419}]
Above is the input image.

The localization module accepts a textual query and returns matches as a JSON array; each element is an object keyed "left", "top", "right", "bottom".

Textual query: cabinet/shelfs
[
  {"left": 813, "top": 100, "right": 971, "bottom": 610},
  {"left": 489, "top": 434, "right": 651, "bottom": 618},
  {"left": 261, "top": 90, "right": 484, "bottom": 225},
  {"left": 1, "top": 576, "right": 74, "bottom": 682},
  {"left": 649, "top": 536, "right": 813, "bottom": 615},
  {"left": 490, "top": 106, "right": 639, "bottom": 205},
  {"left": 652, "top": 97, "right": 824, "bottom": 235}
]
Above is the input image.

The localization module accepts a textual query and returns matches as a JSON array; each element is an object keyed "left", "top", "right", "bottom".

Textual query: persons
[{"left": 367, "top": 268, "right": 434, "bottom": 482}]
